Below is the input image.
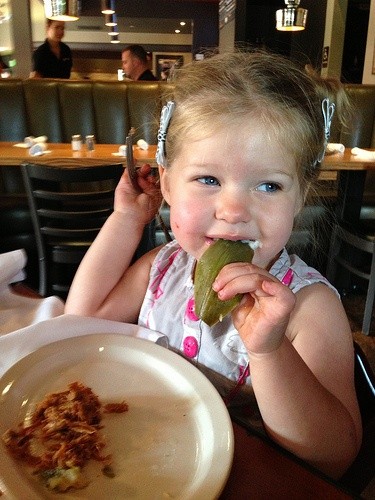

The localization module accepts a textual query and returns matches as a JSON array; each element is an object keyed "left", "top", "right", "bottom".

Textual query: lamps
[
  {"left": 101, "top": 0, "right": 120, "bottom": 43},
  {"left": 43, "top": 0, "right": 80, "bottom": 21},
  {"left": 275, "top": 0, "right": 308, "bottom": 31}
]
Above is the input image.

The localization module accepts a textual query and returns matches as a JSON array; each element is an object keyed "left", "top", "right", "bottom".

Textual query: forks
[{"left": 125, "top": 132, "right": 173, "bottom": 243}]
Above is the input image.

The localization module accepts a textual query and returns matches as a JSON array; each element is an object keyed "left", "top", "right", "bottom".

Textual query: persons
[
  {"left": 122, "top": 46, "right": 155, "bottom": 80},
  {"left": 30, "top": 19, "right": 72, "bottom": 79},
  {"left": 69, "top": 56, "right": 361, "bottom": 480}
]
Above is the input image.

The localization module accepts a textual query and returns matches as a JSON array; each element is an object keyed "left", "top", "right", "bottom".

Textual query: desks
[{"left": 0, "top": 141, "right": 375, "bottom": 224}]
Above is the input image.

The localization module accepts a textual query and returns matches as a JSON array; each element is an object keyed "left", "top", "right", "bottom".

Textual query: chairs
[
  {"left": 326, "top": 224, "right": 375, "bottom": 336},
  {"left": 20, "top": 163, "right": 123, "bottom": 296}
]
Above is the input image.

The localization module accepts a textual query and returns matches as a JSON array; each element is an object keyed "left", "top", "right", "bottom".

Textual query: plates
[{"left": 1, "top": 332, "right": 235, "bottom": 500}]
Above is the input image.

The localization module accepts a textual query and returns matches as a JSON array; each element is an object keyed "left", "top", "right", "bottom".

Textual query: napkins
[{"left": 0, "top": 249, "right": 168, "bottom": 377}]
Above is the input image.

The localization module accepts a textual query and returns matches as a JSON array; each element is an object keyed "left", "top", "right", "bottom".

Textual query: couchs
[{"left": 0, "top": 78, "right": 375, "bottom": 288}]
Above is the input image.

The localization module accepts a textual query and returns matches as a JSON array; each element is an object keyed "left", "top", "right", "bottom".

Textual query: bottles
[
  {"left": 86, "top": 135, "right": 94, "bottom": 149},
  {"left": 71, "top": 135, "right": 81, "bottom": 151}
]
